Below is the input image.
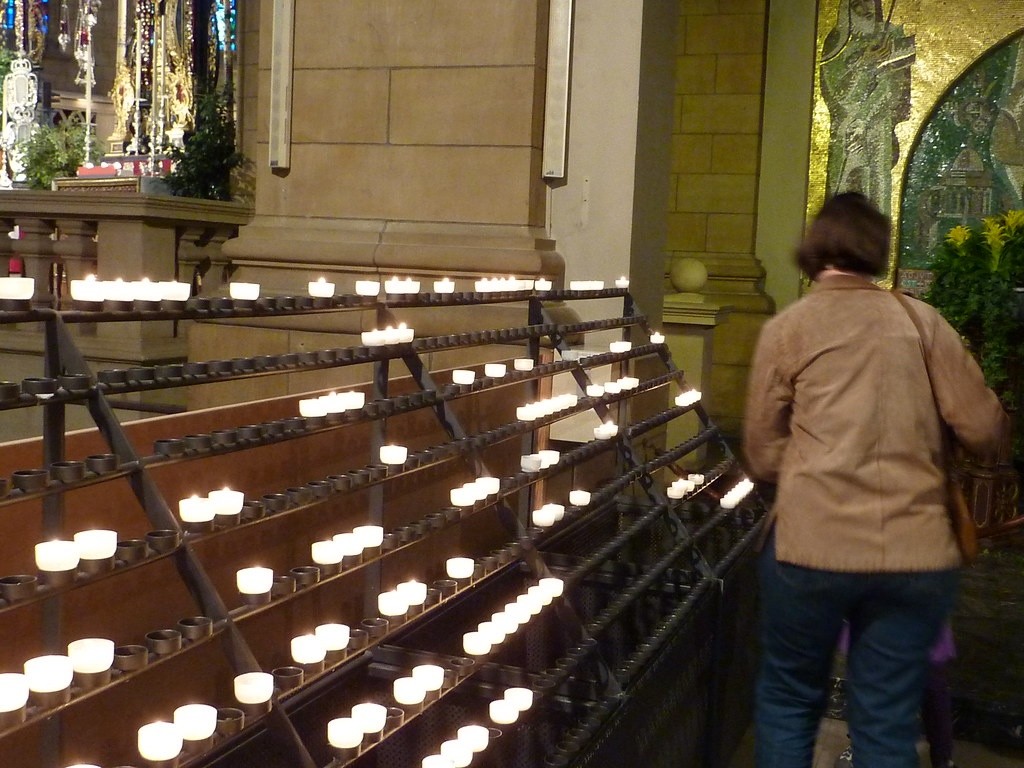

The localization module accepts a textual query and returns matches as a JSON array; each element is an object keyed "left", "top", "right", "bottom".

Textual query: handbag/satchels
[{"left": 946, "top": 468, "right": 976, "bottom": 559}]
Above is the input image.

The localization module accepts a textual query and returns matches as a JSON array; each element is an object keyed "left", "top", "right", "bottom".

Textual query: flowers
[{"left": 920, "top": 211, "right": 1024, "bottom": 461}]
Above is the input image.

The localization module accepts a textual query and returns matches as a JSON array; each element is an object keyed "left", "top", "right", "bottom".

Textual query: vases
[{"left": 947, "top": 356, "right": 1020, "bottom": 538}]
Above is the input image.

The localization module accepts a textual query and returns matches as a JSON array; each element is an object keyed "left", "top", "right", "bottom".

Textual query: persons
[
  {"left": 744, "top": 194, "right": 1008, "bottom": 768},
  {"left": 836, "top": 617, "right": 957, "bottom": 768}
]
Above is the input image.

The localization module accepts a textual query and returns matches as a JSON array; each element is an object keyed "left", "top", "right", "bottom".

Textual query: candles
[
  {"left": 291, "top": 623, "right": 350, "bottom": 663},
  {"left": 34, "top": 530, "right": 117, "bottom": 572},
  {"left": 0, "top": 274, "right": 639, "bottom": 299},
  {"left": 675, "top": 389, "right": 702, "bottom": 406},
  {"left": 421, "top": 726, "right": 489, "bottom": 768},
  {"left": 453, "top": 370, "right": 475, "bottom": 384},
  {"left": 610, "top": 341, "right": 632, "bottom": 353},
  {"left": 361, "top": 322, "right": 415, "bottom": 346},
  {"left": 463, "top": 578, "right": 563, "bottom": 656},
  {"left": 650, "top": 332, "right": 665, "bottom": 344},
  {"left": 562, "top": 351, "right": 579, "bottom": 359},
  {"left": 394, "top": 664, "right": 445, "bottom": 705},
  {"left": 514, "top": 359, "right": 534, "bottom": 371},
  {"left": 379, "top": 581, "right": 427, "bottom": 616},
  {"left": 720, "top": 479, "right": 754, "bottom": 509},
  {"left": 237, "top": 567, "right": 273, "bottom": 594},
  {"left": 179, "top": 487, "right": 244, "bottom": 523},
  {"left": 327, "top": 702, "right": 388, "bottom": 747},
  {"left": 234, "top": 672, "right": 273, "bottom": 704},
  {"left": 667, "top": 474, "right": 704, "bottom": 499},
  {"left": 138, "top": 703, "right": 217, "bottom": 761},
  {"left": 485, "top": 364, "right": 506, "bottom": 377},
  {"left": 447, "top": 558, "right": 475, "bottom": 578},
  {"left": 587, "top": 377, "right": 639, "bottom": 397},
  {"left": 299, "top": 391, "right": 366, "bottom": 417},
  {"left": 490, "top": 688, "right": 534, "bottom": 724},
  {"left": 533, "top": 490, "right": 591, "bottom": 527},
  {"left": 594, "top": 420, "right": 618, "bottom": 440},
  {"left": 380, "top": 444, "right": 408, "bottom": 465},
  {"left": 0, "top": 638, "right": 114, "bottom": 712},
  {"left": 450, "top": 476, "right": 500, "bottom": 507},
  {"left": 521, "top": 450, "right": 559, "bottom": 472},
  {"left": 312, "top": 525, "right": 384, "bottom": 564},
  {"left": 516, "top": 393, "right": 578, "bottom": 421}
]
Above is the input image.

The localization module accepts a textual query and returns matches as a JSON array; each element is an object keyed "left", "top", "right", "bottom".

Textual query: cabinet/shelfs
[{"left": 0, "top": 288, "right": 775, "bottom": 768}]
